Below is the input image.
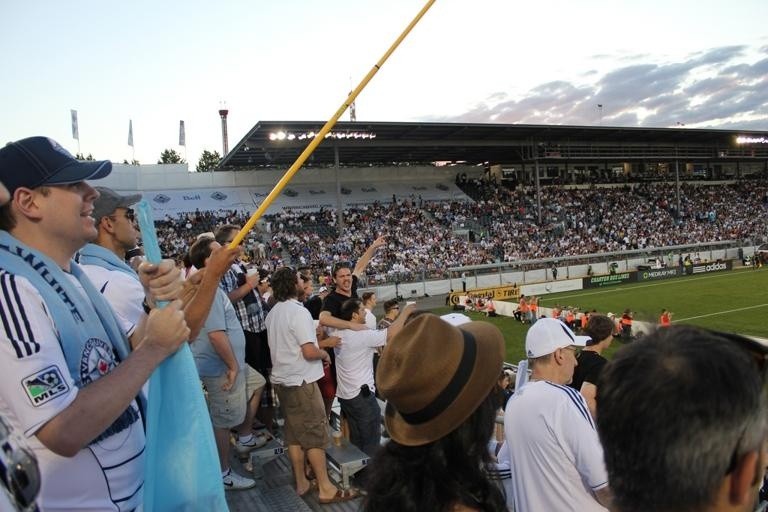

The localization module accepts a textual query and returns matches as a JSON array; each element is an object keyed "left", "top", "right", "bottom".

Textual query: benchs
[{"left": 276, "top": 415, "right": 368, "bottom": 492}]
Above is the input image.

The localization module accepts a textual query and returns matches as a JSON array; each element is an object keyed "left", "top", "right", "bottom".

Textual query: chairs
[{"left": 146, "top": 201, "right": 438, "bottom": 291}]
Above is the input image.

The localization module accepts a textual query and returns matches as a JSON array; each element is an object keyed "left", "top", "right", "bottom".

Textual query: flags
[
  {"left": 70, "top": 108, "right": 81, "bottom": 141},
  {"left": 126, "top": 119, "right": 138, "bottom": 148},
  {"left": 178, "top": 119, "right": 187, "bottom": 147}
]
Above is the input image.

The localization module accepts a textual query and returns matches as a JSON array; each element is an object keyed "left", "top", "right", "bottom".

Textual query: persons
[
  {"left": 125, "top": 156, "right": 767, "bottom": 511},
  {"left": 80, "top": 183, "right": 242, "bottom": 512},
  {"left": 1, "top": 136, "right": 191, "bottom": 512}
]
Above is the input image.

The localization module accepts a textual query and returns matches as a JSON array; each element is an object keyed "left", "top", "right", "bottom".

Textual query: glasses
[
  {"left": 109, "top": 208, "right": 135, "bottom": 222},
  {"left": 331, "top": 261, "right": 352, "bottom": 273}
]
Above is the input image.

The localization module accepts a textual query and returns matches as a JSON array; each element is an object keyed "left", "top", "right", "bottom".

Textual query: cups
[
  {"left": 405, "top": 299, "right": 416, "bottom": 314},
  {"left": 247, "top": 268, "right": 257, "bottom": 278},
  {"left": 332, "top": 431, "right": 343, "bottom": 447}
]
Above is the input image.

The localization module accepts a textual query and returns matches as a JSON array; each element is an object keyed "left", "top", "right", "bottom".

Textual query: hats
[
  {"left": 524, "top": 316, "right": 592, "bottom": 360},
  {"left": 376, "top": 311, "right": 505, "bottom": 445},
  {"left": 1, "top": 135, "right": 113, "bottom": 201},
  {"left": 91, "top": 186, "right": 142, "bottom": 224}
]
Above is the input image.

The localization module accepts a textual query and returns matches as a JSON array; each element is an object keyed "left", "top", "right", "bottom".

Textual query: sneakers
[
  {"left": 223, "top": 467, "right": 255, "bottom": 492},
  {"left": 236, "top": 435, "right": 266, "bottom": 452}
]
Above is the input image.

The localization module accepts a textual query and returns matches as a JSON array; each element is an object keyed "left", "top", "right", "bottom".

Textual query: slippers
[
  {"left": 300, "top": 479, "right": 318, "bottom": 496},
  {"left": 319, "top": 487, "right": 361, "bottom": 503}
]
